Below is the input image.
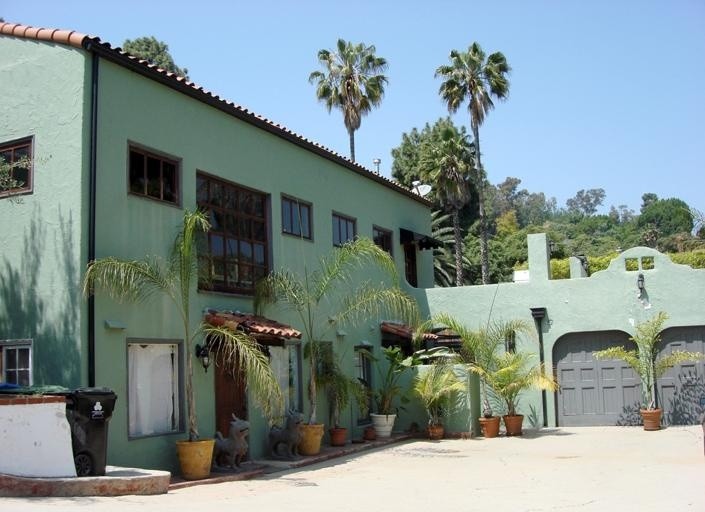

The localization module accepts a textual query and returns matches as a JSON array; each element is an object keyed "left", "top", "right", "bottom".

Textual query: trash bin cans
[{"left": 0, "top": 382, "right": 118, "bottom": 477}]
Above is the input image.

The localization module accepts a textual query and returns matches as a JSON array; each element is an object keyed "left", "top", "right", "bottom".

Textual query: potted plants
[
  {"left": 251, "top": 231, "right": 420, "bottom": 457},
  {"left": 304, "top": 340, "right": 365, "bottom": 446},
  {"left": 591, "top": 310, "right": 705, "bottom": 431},
  {"left": 408, "top": 364, "right": 460, "bottom": 441},
  {"left": 465, "top": 355, "right": 560, "bottom": 437},
  {"left": 410, "top": 313, "right": 526, "bottom": 439},
  {"left": 357, "top": 344, "right": 460, "bottom": 437},
  {"left": 80, "top": 206, "right": 290, "bottom": 481}
]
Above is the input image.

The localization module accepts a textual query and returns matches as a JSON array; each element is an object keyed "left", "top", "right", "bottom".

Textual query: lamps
[{"left": 637, "top": 278, "right": 644, "bottom": 298}]
